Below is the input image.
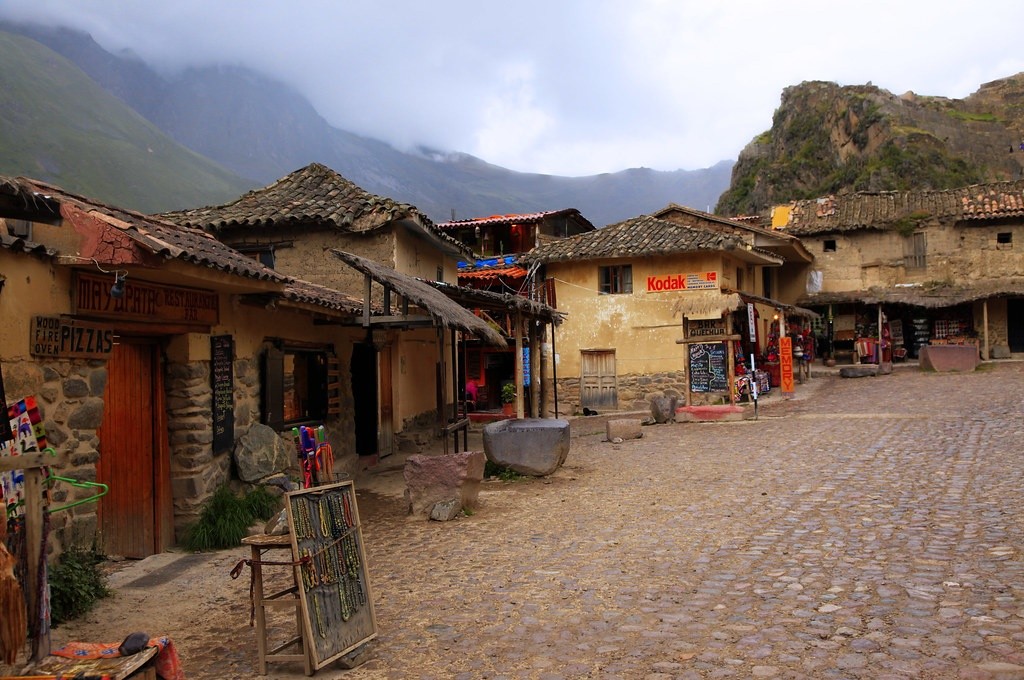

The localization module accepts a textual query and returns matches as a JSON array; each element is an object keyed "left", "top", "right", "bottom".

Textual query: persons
[{"left": 466, "top": 376, "right": 480, "bottom": 411}]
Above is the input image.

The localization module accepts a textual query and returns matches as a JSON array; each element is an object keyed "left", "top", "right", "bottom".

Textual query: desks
[{"left": 241, "top": 534, "right": 311, "bottom": 676}]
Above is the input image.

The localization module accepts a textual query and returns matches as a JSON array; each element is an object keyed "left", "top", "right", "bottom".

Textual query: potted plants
[{"left": 501, "top": 382, "right": 516, "bottom": 416}]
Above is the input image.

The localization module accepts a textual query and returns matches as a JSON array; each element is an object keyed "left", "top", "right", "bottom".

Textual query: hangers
[{"left": 7, "top": 448, "right": 108, "bottom": 519}]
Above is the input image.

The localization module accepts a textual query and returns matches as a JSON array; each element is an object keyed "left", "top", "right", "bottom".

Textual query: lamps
[{"left": 111, "top": 270, "right": 129, "bottom": 298}]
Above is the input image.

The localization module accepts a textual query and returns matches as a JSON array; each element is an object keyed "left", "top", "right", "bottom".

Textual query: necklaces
[{"left": 291, "top": 490, "right": 366, "bottom": 637}]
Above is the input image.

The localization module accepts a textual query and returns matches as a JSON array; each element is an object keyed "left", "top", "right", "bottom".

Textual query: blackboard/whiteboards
[
  {"left": 211, "top": 333, "right": 234, "bottom": 451},
  {"left": 688, "top": 343, "right": 728, "bottom": 392}
]
